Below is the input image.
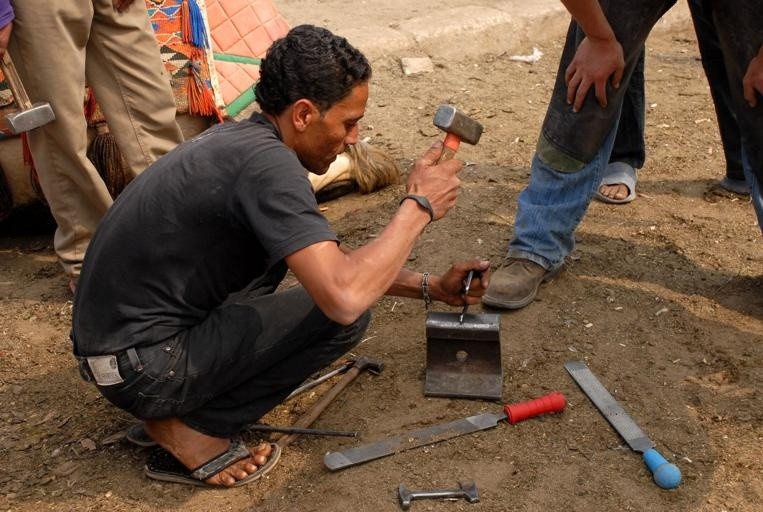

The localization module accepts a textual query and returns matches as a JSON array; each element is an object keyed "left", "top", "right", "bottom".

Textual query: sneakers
[{"left": 481, "top": 258, "right": 567, "bottom": 310}]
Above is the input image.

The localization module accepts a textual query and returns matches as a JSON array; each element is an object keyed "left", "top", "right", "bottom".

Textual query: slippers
[
  {"left": 143, "top": 434, "right": 282, "bottom": 488},
  {"left": 594, "top": 161, "right": 639, "bottom": 204},
  {"left": 126, "top": 421, "right": 158, "bottom": 447}
]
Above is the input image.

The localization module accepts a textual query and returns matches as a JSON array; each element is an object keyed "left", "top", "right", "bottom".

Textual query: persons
[
  {"left": 593, "top": 2, "right": 751, "bottom": 203},
  {"left": 0, "top": 1, "right": 16, "bottom": 62},
  {"left": 70, "top": 24, "right": 493, "bottom": 490},
  {"left": 8, "top": 0, "right": 186, "bottom": 300},
  {"left": 481, "top": 1, "right": 763, "bottom": 310}
]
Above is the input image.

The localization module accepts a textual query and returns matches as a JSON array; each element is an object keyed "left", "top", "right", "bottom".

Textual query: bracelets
[
  {"left": 421, "top": 271, "right": 432, "bottom": 311},
  {"left": 400, "top": 192, "right": 434, "bottom": 225}
]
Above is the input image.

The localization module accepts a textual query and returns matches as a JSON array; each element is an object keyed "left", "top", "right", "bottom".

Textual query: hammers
[
  {"left": 433, "top": 105, "right": 483, "bottom": 166},
  {"left": 0, "top": 49, "right": 56, "bottom": 135},
  {"left": 276, "top": 357, "right": 384, "bottom": 450}
]
